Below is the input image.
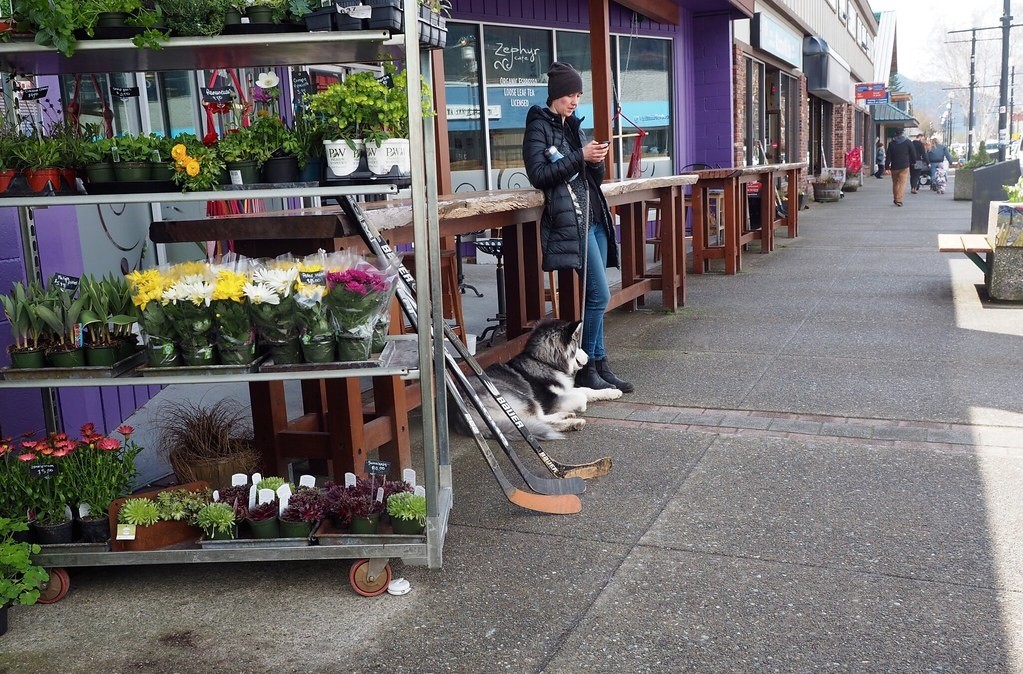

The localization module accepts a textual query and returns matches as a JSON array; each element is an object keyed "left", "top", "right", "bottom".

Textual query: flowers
[
  {"left": 124, "top": 249, "right": 404, "bottom": 337},
  {"left": 253, "top": 71, "right": 295, "bottom": 155},
  {"left": 0, "top": 422, "right": 146, "bottom": 517},
  {"left": 173, "top": 132, "right": 229, "bottom": 191}
]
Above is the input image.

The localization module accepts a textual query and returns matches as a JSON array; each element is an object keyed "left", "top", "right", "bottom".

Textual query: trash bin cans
[{"left": 970, "top": 158, "right": 1021, "bottom": 234}]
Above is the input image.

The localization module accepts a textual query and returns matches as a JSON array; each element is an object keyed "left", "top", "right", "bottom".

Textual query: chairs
[{"left": 677, "top": 163, "right": 715, "bottom": 208}]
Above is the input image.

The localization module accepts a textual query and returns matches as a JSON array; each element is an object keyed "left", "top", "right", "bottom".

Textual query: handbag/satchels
[
  {"left": 875, "top": 165, "right": 878, "bottom": 173},
  {"left": 914, "top": 160, "right": 922, "bottom": 170}
]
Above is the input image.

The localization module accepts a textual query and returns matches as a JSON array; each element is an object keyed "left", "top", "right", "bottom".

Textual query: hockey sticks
[{"left": 336, "top": 195, "right": 615, "bottom": 518}]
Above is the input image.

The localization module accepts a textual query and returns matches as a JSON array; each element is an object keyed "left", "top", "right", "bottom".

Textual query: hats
[{"left": 547, "top": 62, "right": 583, "bottom": 100}]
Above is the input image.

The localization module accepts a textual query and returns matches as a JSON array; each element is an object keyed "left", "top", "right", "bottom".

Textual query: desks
[
  {"left": 690, "top": 162, "right": 809, "bottom": 274},
  {"left": 150, "top": 174, "right": 686, "bottom": 477}
]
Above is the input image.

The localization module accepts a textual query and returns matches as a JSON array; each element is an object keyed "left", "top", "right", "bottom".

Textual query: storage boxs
[{"left": 334, "top": 0, "right": 448, "bottom": 49}]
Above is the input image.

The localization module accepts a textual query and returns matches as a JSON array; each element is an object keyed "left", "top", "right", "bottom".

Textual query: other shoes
[
  {"left": 941, "top": 191, "right": 944, "bottom": 194},
  {"left": 933, "top": 186, "right": 937, "bottom": 191},
  {"left": 911, "top": 188, "right": 918, "bottom": 193},
  {"left": 893, "top": 200, "right": 897, "bottom": 204},
  {"left": 937, "top": 191, "right": 940, "bottom": 194},
  {"left": 897, "top": 202, "right": 903, "bottom": 206}
]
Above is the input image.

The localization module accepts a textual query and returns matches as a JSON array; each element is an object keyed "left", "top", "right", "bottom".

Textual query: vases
[
  {"left": 265, "top": 153, "right": 294, "bottom": 183},
  {"left": 8, "top": 510, "right": 111, "bottom": 544},
  {"left": 147, "top": 316, "right": 391, "bottom": 366}
]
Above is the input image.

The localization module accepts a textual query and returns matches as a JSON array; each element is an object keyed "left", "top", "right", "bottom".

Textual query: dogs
[{"left": 448, "top": 317, "right": 624, "bottom": 441}]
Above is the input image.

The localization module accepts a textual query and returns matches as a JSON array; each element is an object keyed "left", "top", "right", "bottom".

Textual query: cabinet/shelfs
[{"left": 0, "top": 0, "right": 455, "bottom": 597}]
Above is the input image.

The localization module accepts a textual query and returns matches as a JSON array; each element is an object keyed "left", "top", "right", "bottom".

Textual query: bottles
[{"left": 545, "top": 145, "right": 579, "bottom": 183}]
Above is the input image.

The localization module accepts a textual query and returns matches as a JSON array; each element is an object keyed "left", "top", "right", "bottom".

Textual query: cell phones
[{"left": 600, "top": 141, "right": 610, "bottom": 149}]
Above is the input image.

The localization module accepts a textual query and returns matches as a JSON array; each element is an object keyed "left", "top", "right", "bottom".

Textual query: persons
[
  {"left": 874, "top": 128, "right": 953, "bottom": 206},
  {"left": 522, "top": 62, "right": 636, "bottom": 394}
]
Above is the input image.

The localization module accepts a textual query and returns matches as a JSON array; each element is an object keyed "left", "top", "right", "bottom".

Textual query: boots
[
  {"left": 574, "top": 358, "right": 616, "bottom": 390},
  {"left": 595, "top": 359, "right": 635, "bottom": 393}
]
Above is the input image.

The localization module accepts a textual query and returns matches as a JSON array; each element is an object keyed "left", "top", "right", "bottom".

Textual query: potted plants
[
  {"left": 417, "top": 0, "right": 422, "bottom": 16},
  {"left": 799, "top": 189, "right": 809, "bottom": 211},
  {"left": 151, "top": 385, "right": 260, "bottom": 485},
  {"left": 986, "top": 175, "right": 1023, "bottom": 251},
  {"left": 113, "top": 129, "right": 174, "bottom": 181},
  {"left": 80, "top": 138, "right": 112, "bottom": 181},
  {"left": 0, "top": 272, "right": 138, "bottom": 368},
  {"left": 59, "top": 137, "right": 77, "bottom": 189},
  {"left": 19, "top": 128, "right": 61, "bottom": 191},
  {"left": 953, "top": 140, "right": 992, "bottom": 200},
  {"left": 422, "top": 0, "right": 431, "bottom": 20},
  {"left": 0, "top": 130, "right": 18, "bottom": 193},
  {"left": 196, "top": 473, "right": 427, "bottom": 541},
  {"left": 300, "top": 52, "right": 437, "bottom": 176},
  {"left": 291, "top": 0, "right": 334, "bottom": 30},
  {"left": 0, "top": 518, "right": 49, "bottom": 636},
  {"left": 110, "top": 480, "right": 212, "bottom": 551},
  {"left": 220, "top": 128, "right": 258, "bottom": 183},
  {"left": 439, "top": 8, "right": 448, "bottom": 28},
  {"left": 812, "top": 171, "right": 843, "bottom": 203},
  {"left": 430, "top": 0, "right": 440, "bottom": 26},
  {"left": 97, "top": 0, "right": 286, "bottom": 27}
]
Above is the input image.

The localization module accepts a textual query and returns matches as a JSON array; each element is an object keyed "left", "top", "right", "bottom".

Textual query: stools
[
  {"left": 709, "top": 188, "right": 724, "bottom": 246},
  {"left": 472, "top": 238, "right": 506, "bottom": 348},
  {"left": 644, "top": 198, "right": 661, "bottom": 263},
  {"left": 403, "top": 250, "right": 467, "bottom": 347}
]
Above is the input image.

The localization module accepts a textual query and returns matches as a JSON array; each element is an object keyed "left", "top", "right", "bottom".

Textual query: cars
[{"left": 984, "top": 140, "right": 999, "bottom": 155}]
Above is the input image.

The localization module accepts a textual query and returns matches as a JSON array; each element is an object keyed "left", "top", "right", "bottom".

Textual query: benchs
[{"left": 937, "top": 233, "right": 993, "bottom": 275}]
